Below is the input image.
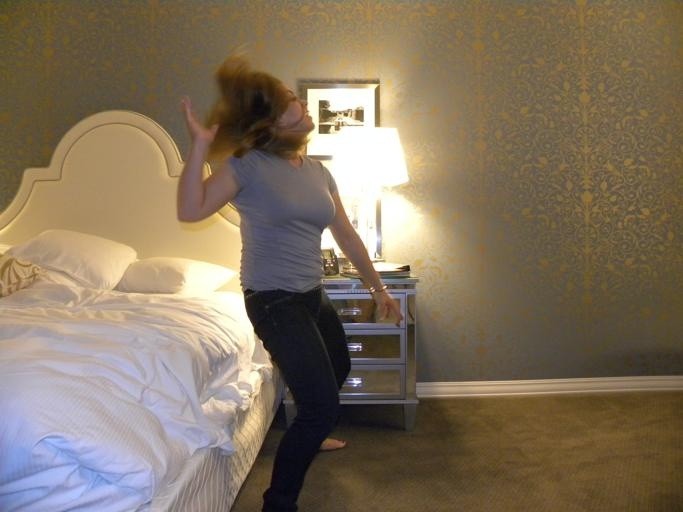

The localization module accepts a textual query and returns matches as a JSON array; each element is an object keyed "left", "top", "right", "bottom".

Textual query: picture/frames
[{"left": 299, "top": 79, "right": 381, "bottom": 161}]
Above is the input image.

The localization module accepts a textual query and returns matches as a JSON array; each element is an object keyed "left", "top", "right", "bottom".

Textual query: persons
[{"left": 175, "top": 41, "right": 404, "bottom": 512}]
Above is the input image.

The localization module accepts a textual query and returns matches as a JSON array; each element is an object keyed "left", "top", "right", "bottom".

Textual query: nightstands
[{"left": 284, "top": 273, "right": 418, "bottom": 437}]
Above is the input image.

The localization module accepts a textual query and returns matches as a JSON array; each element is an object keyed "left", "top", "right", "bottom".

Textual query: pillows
[{"left": 0, "top": 228, "right": 241, "bottom": 298}]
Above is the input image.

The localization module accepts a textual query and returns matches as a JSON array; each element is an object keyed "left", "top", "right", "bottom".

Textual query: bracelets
[{"left": 369, "top": 284, "right": 387, "bottom": 295}]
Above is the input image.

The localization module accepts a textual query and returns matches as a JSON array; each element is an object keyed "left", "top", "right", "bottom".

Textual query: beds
[{"left": 0, "top": 109, "right": 285, "bottom": 512}]
